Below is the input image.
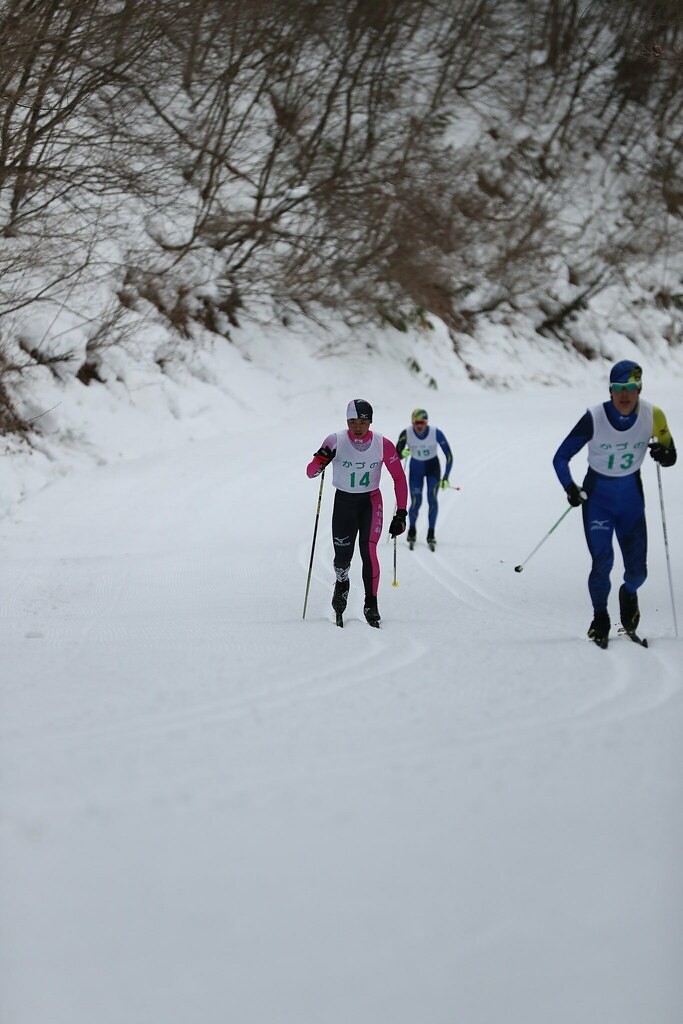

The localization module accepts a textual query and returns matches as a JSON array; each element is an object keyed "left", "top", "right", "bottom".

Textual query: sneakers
[
  {"left": 407, "top": 527, "right": 416, "bottom": 550},
  {"left": 427, "top": 529, "right": 436, "bottom": 551},
  {"left": 363, "top": 596, "right": 381, "bottom": 628},
  {"left": 619, "top": 583, "right": 640, "bottom": 634},
  {"left": 587, "top": 609, "right": 611, "bottom": 649},
  {"left": 331, "top": 578, "right": 350, "bottom": 628}
]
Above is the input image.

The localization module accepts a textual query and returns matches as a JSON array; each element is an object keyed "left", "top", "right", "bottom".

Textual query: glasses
[{"left": 611, "top": 383, "right": 636, "bottom": 392}]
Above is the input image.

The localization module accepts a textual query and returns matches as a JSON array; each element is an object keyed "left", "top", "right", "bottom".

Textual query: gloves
[
  {"left": 313, "top": 445, "right": 336, "bottom": 470},
  {"left": 437, "top": 479, "right": 449, "bottom": 488},
  {"left": 647, "top": 442, "right": 667, "bottom": 461},
  {"left": 389, "top": 509, "right": 408, "bottom": 539},
  {"left": 401, "top": 448, "right": 413, "bottom": 458},
  {"left": 564, "top": 483, "right": 590, "bottom": 507}
]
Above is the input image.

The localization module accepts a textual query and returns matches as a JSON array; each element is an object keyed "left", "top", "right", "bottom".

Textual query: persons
[
  {"left": 395, "top": 408, "right": 453, "bottom": 544},
  {"left": 552, "top": 359, "right": 676, "bottom": 638},
  {"left": 306, "top": 399, "right": 408, "bottom": 622}
]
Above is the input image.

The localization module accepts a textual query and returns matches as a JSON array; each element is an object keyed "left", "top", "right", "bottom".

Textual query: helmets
[
  {"left": 610, "top": 360, "right": 642, "bottom": 382},
  {"left": 411, "top": 408, "right": 428, "bottom": 421},
  {"left": 346, "top": 399, "right": 373, "bottom": 423}
]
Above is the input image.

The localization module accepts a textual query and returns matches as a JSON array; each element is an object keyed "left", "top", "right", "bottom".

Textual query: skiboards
[
  {"left": 595, "top": 632, "right": 648, "bottom": 649},
  {"left": 409, "top": 540, "right": 435, "bottom": 552},
  {"left": 336, "top": 611, "right": 379, "bottom": 629}
]
